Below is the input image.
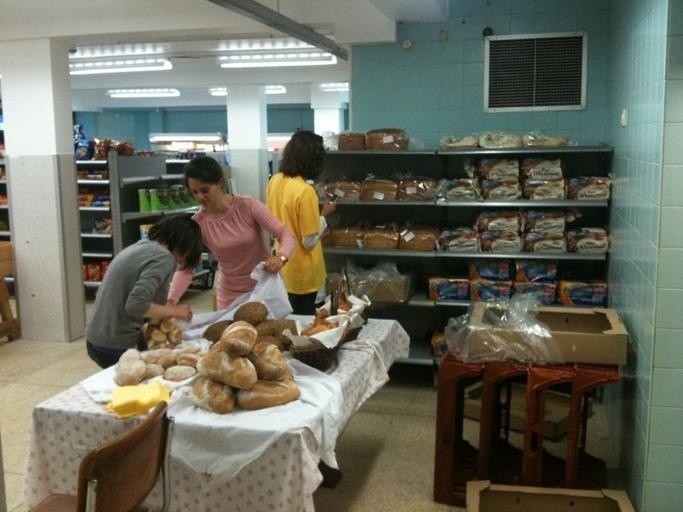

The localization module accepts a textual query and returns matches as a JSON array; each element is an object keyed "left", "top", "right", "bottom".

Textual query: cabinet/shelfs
[
  {"left": 0, "top": 148, "right": 278, "bottom": 286},
  {"left": 312, "top": 147, "right": 613, "bottom": 366}
]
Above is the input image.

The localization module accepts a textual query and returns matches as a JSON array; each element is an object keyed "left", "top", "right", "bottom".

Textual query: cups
[
  {"left": 136, "top": 185, "right": 198, "bottom": 211},
  {"left": 138, "top": 224, "right": 153, "bottom": 241}
]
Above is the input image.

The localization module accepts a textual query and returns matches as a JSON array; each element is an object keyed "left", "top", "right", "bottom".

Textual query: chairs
[{"left": 28, "top": 401, "right": 175, "bottom": 512}]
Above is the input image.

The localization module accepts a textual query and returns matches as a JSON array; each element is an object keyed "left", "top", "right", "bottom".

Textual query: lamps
[
  {"left": 69, "top": 52, "right": 349, "bottom": 98},
  {"left": 266, "top": 133, "right": 295, "bottom": 141},
  {"left": 148, "top": 131, "right": 224, "bottom": 144}
]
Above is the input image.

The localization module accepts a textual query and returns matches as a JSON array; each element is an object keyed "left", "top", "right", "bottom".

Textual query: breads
[
  {"left": 114, "top": 301, "right": 300, "bottom": 415},
  {"left": 323, "top": 156, "right": 610, "bottom": 201},
  {"left": 320, "top": 206, "right": 608, "bottom": 254},
  {"left": 312, "top": 128, "right": 611, "bottom": 150},
  {"left": 302, "top": 308, "right": 340, "bottom": 336},
  {"left": 339, "top": 287, "right": 353, "bottom": 311}
]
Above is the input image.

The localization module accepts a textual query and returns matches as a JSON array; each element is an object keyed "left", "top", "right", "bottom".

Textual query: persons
[
  {"left": 265, "top": 130, "right": 336, "bottom": 316},
  {"left": 86, "top": 214, "right": 204, "bottom": 369},
  {"left": 164, "top": 155, "right": 297, "bottom": 311}
]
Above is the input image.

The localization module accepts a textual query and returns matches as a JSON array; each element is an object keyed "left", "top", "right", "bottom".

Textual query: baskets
[
  {"left": 330, "top": 288, "right": 363, "bottom": 343},
  {"left": 341, "top": 266, "right": 369, "bottom": 324},
  {"left": 288, "top": 343, "right": 337, "bottom": 372}
]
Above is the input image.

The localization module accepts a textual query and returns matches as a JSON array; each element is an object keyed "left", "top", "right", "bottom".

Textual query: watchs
[{"left": 276, "top": 253, "right": 288, "bottom": 265}]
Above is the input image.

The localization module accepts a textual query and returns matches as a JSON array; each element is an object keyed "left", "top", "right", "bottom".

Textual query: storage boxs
[
  {"left": 463, "top": 378, "right": 593, "bottom": 440},
  {"left": 465, "top": 480, "right": 635, "bottom": 512},
  {"left": 326, "top": 266, "right": 421, "bottom": 305},
  {"left": 467, "top": 295, "right": 628, "bottom": 366}
]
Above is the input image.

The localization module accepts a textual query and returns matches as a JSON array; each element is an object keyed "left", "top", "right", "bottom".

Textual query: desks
[{"left": 21, "top": 314, "right": 412, "bottom": 512}]
